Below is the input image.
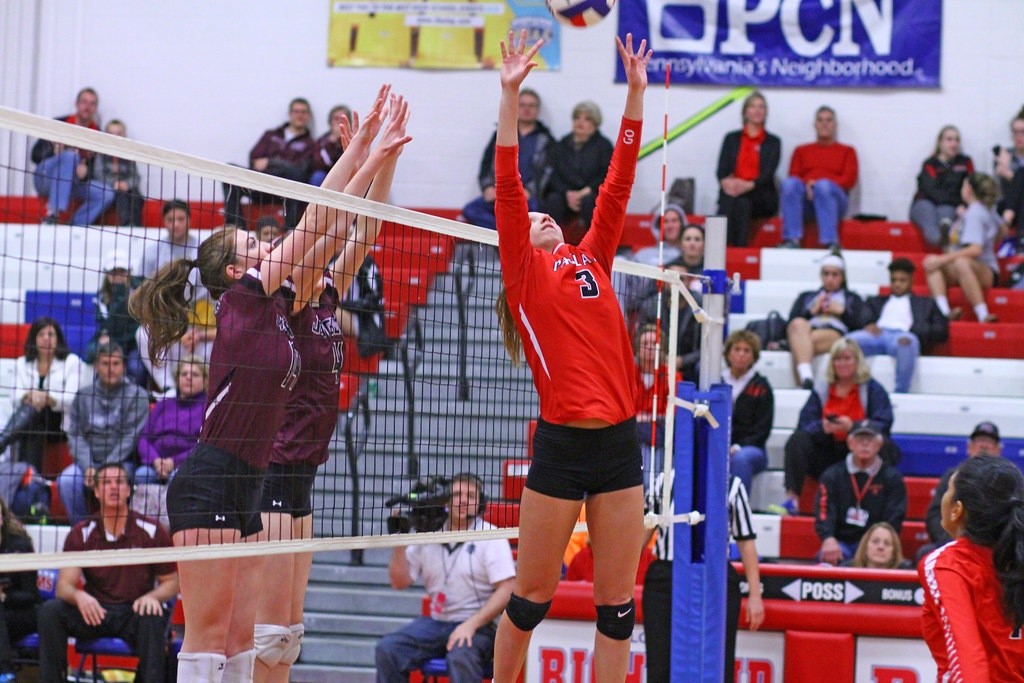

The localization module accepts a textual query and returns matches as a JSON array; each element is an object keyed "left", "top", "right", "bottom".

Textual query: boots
[
  {"left": 0, "top": 403, "right": 40, "bottom": 457},
  {"left": 19, "top": 435, "right": 43, "bottom": 473}
]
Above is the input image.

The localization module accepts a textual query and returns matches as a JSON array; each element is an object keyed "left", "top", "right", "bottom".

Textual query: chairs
[
  {"left": 75, "top": 592, "right": 177, "bottom": 683},
  {"left": 0, "top": 568, "right": 58, "bottom": 683}
]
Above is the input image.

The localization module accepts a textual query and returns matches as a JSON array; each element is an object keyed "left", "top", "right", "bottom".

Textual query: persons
[
  {"left": 540, "top": 97, "right": 614, "bottom": 233},
  {"left": 715, "top": 92, "right": 779, "bottom": 248},
  {"left": 0, "top": 85, "right": 413, "bottom": 683},
  {"left": 778, "top": 105, "right": 858, "bottom": 249},
  {"left": 561, "top": 103, "right": 1024, "bottom": 683},
  {"left": 370, "top": 472, "right": 516, "bottom": 683},
  {"left": 494, "top": 27, "right": 652, "bottom": 683},
  {"left": 463, "top": 88, "right": 555, "bottom": 231}
]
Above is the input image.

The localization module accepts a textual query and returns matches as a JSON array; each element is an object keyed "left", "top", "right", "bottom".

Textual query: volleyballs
[{"left": 544, "top": 0, "right": 616, "bottom": 30}]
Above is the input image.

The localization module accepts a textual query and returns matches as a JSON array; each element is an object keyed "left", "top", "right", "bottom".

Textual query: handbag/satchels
[
  {"left": 746, "top": 310, "right": 789, "bottom": 349},
  {"left": 667, "top": 177, "right": 695, "bottom": 214}
]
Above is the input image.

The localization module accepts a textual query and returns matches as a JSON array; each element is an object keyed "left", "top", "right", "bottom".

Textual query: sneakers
[{"left": 767, "top": 497, "right": 800, "bottom": 516}]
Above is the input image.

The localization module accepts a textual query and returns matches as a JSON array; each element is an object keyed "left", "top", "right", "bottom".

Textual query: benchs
[{"left": 0, "top": 195, "right": 1024, "bottom": 683}]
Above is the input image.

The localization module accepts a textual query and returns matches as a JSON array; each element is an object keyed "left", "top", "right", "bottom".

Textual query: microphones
[{"left": 385, "top": 494, "right": 404, "bottom": 507}]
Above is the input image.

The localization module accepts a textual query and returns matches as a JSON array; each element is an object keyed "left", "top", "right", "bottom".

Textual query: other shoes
[
  {"left": 777, "top": 238, "right": 801, "bottom": 248},
  {"left": 984, "top": 314, "right": 998, "bottom": 323},
  {"left": 941, "top": 224, "right": 951, "bottom": 246},
  {"left": 948, "top": 307, "right": 963, "bottom": 320},
  {"left": 41, "top": 214, "right": 57, "bottom": 224},
  {"left": 996, "top": 237, "right": 1017, "bottom": 257},
  {"left": 825, "top": 243, "right": 839, "bottom": 250}
]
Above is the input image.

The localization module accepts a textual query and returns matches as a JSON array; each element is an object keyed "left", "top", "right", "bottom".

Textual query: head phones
[{"left": 454, "top": 472, "right": 488, "bottom": 512}]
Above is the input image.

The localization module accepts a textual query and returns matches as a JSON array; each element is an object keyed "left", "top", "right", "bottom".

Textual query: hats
[
  {"left": 257, "top": 215, "right": 279, "bottom": 230},
  {"left": 853, "top": 419, "right": 879, "bottom": 435},
  {"left": 104, "top": 251, "right": 132, "bottom": 272},
  {"left": 975, "top": 422, "right": 1000, "bottom": 441}
]
